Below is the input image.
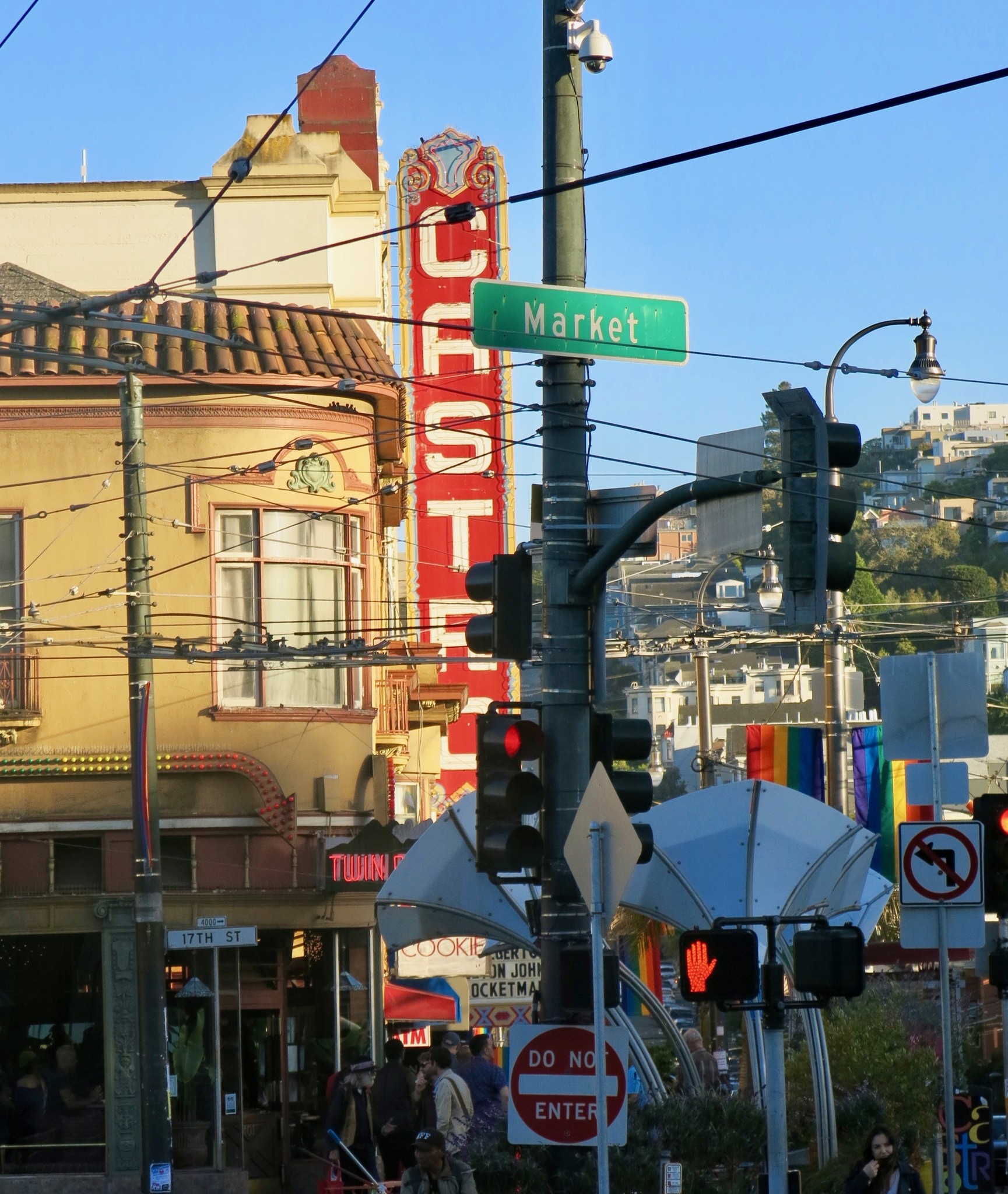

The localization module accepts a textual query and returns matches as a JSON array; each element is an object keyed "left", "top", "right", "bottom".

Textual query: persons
[
  {"left": 627, "top": 1065, "right": 650, "bottom": 1109},
  {"left": 719, "top": 1073, "right": 730, "bottom": 1096},
  {"left": 0, "top": 1019, "right": 105, "bottom": 1146},
  {"left": 671, "top": 1029, "right": 721, "bottom": 1097},
  {"left": 841, "top": 1125, "right": 927, "bottom": 1194},
  {"left": 325, "top": 1031, "right": 509, "bottom": 1194},
  {"left": 399, "top": 1128, "right": 478, "bottom": 1194}
]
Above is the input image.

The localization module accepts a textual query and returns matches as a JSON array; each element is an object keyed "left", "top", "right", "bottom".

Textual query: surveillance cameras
[{"left": 577, "top": 31, "right": 614, "bottom": 75}]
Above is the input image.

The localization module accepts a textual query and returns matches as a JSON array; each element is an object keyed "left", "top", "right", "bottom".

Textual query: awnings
[{"left": 383, "top": 983, "right": 457, "bottom": 1025}]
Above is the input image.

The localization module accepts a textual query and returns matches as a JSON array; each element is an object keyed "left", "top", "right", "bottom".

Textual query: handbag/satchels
[{"left": 317, "top": 1159, "right": 344, "bottom": 1194}]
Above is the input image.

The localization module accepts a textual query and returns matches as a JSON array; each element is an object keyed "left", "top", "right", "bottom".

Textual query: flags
[
  {"left": 745, "top": 724, "right": 826, "bottom": 805},
  {"left": 851, "top": 724, "right": 936, "bottom": 881}
]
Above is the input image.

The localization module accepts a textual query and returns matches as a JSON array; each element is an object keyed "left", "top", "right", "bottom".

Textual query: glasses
[
  {"left": 488, "top": 1044, "right": 496, "bottom": 1050},
  {"left": 421, "top": 1062, "right": 427, "bottom": 1068}
]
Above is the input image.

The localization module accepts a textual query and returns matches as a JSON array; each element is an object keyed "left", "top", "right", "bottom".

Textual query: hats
[
  {"left": 408, "top": 1127, "right": 446, "bottom": 1151},
  {"left": 347, "top": 1054, "right": 380, "bottom": 1073},
  {"left": 443, "top": 1031, "right": 461, "bottom": 1045}
]
